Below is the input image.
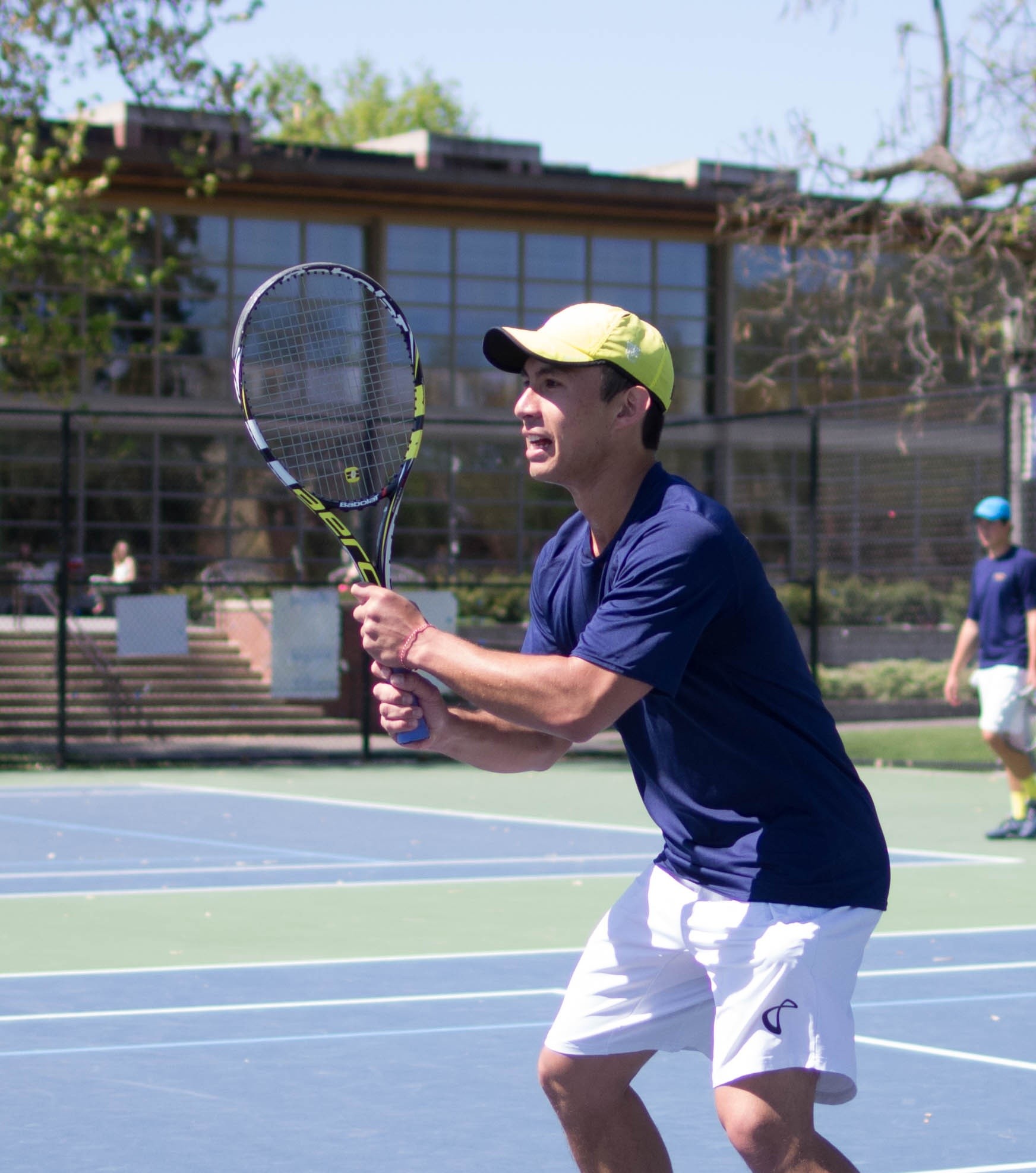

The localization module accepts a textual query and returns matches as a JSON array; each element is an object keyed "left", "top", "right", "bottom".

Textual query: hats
[
  {"left": 973, "top": 496, "right": 1011, "bottom": 521},
  {"left": 482, "top": 302, "right": 674, "bottom": 411}
]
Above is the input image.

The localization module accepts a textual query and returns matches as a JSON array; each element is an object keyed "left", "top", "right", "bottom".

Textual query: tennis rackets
[
  {"left": 995, "top": 684, "right": 1036, "bottom": 756},
  {"left": 230, "top": 259, "right": 431, "bottom": 745}
]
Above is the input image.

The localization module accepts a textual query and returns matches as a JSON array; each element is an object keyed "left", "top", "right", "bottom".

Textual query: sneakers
[
  {"left": 987, "top": 817, "right": 1017, "bottom": 839},
  {"left": 1018, "top": 806, "right": 1036, "bottom": 838}
]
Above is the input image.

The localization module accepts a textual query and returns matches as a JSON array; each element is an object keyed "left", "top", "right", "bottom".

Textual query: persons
[
  {"left": 942, "top": 494, "right": 1036, "bottom": 840},
  {"left": 347, "top": 302, "right": 891, "bottom": 1173},
  {"left": 6, "top": 541, "right": 73, "bottom": 617},
  {"left": 89, "top": 541, "right": 138, "bottom": 613}
]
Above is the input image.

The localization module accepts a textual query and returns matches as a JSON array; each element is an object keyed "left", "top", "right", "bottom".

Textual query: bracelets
[{"left": 399, "top": 622, "right": 439, "bottom": 671}]
